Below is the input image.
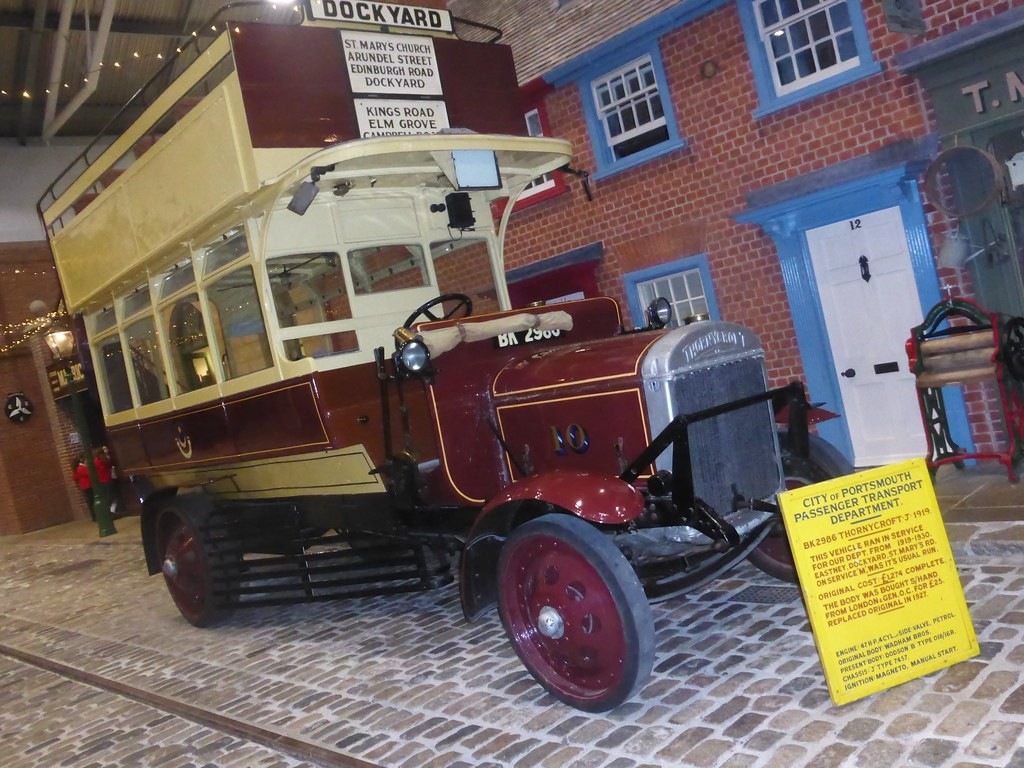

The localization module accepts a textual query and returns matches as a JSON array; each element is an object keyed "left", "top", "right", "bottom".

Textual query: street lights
[{"left": 43, "top": 317, "right": 120, "bottom": 539}]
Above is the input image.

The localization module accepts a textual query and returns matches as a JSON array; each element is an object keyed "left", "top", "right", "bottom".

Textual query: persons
[
  {"left": 94, "top": 445, "right": 124, "bottom": 519},
  {"left": 72, "top": 451, "right": 96, "bottom": 521}
]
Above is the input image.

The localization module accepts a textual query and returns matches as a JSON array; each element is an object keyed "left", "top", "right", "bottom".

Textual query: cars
[{"left": 36, "top": 0, "right": 856, "bottom": 713}]
